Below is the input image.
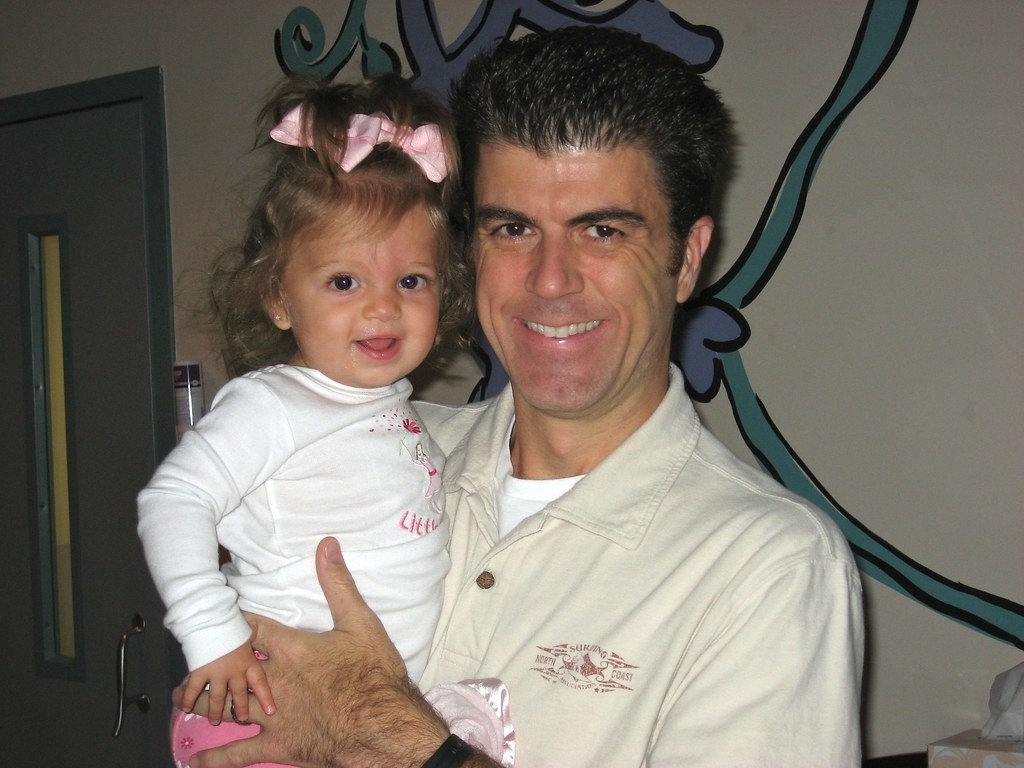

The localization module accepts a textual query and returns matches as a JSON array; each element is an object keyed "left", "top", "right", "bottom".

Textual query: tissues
[{"left": 927, "top": 661, "right": 1023, "bottom": 767}]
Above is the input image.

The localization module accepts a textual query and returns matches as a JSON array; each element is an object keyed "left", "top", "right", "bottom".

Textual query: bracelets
[{"left": 420, "top": 734, "right": 473, "bottom": 768}]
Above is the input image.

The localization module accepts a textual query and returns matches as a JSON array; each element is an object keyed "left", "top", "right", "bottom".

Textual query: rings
[{"left": 231, "top": 700, "right": 247, "bottom": 724}]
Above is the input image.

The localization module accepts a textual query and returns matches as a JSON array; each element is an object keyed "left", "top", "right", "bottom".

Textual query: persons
[
  {"left": 168, "top": 23, "right": 867, "bottom": 768},
  {"left": 134, "top": 75, "right": 476, "bottom": 768}
]
